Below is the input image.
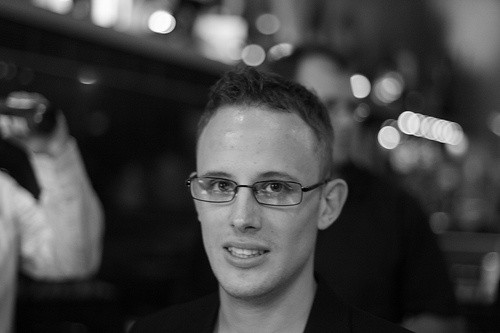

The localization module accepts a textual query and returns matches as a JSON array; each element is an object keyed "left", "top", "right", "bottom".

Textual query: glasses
[{"left": 184, "top": 173, "right": 331, "bottom": 207}]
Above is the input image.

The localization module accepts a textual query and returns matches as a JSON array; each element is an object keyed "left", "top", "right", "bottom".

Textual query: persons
[
  {"left": 125, "top": 57, "right": 418, "bottom": 332},
  {"left": 0, "top": 89, "right": 107, "bottom": 332}
]
